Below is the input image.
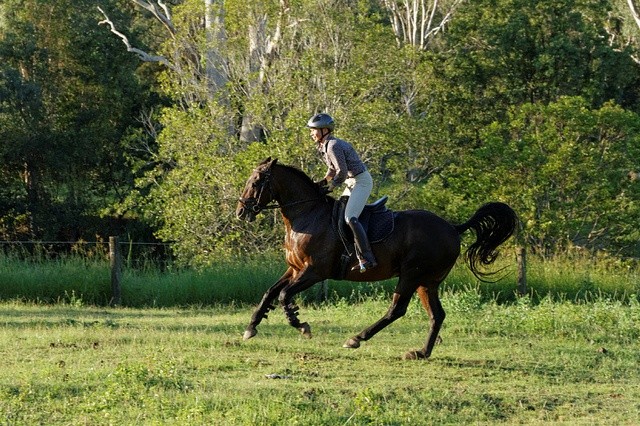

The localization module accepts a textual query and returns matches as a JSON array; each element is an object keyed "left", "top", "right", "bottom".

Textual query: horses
[{"left": 235, "top": 155, "right": 518, "bottom": 360}]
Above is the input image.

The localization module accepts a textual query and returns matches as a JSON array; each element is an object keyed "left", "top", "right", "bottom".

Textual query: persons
[{"left": 306, "top": 113, "right": 379, "bottom": 272}]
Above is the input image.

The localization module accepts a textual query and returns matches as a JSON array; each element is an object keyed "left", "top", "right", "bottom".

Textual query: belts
[{"left": 346, "top": 168, "right": 367, "bottom": 179}]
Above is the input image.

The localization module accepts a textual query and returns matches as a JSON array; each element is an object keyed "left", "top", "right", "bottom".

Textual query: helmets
[{"left": 306, "top": 112, "right": 336, "bottom": 144}]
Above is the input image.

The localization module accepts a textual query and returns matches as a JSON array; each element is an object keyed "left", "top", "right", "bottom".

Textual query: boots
[{"left": 347, "top": 217, "right": 379, "bottom": 271}]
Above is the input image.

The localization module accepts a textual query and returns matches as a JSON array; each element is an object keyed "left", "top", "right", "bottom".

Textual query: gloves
[
  {"left": 314, "top": 186, "right": 333, "bottom": 198},
  {"left": 312, "top": 178, "right": 328, "bottom": 186}
]
